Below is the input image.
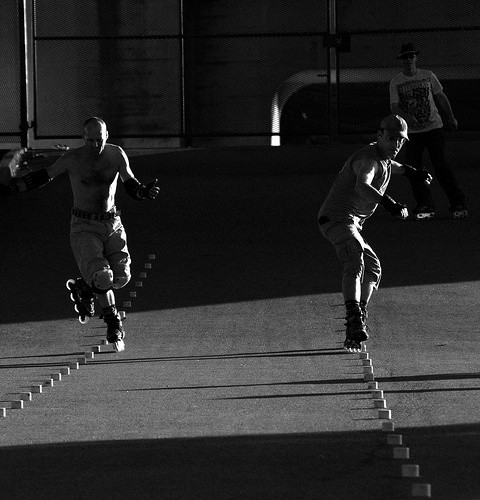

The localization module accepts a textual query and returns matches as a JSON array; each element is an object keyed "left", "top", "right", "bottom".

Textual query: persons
[
  {"left": 0, "top": 117, "right": 161, "bottom": 344},
  {"left": 389, "top": 43, "right": 471, "bottom": 221},
  {"left": 317, "top": 114, "right": 432, "bottom": 349}
]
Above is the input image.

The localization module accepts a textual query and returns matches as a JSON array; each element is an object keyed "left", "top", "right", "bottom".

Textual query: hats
[
  {"left": 396, "top": 43, "right": 420, "bottom": 58},
  {"left": 379, "top": 114, "right": 410, "bottom": 140}
]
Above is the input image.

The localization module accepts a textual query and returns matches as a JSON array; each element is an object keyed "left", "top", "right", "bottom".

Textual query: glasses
[
  {"left": 380, "top": 134, "right": 406, "bottom": 144},
  {"left": 400, "top": 54, "right": 417, "bottom": 60}
]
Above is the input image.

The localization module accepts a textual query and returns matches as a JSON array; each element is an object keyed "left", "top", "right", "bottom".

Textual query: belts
[{"left": 71, "top": 205, "right": 121, "bottom": 220}]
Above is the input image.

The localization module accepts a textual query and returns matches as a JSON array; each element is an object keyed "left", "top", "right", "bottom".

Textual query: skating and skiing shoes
[
  {"left": 448, "top": 201, "right": 469, "bottom": 218},
  {"left": 65, "top": 277, "right": 96, "bottom": 324},
  {"left": 412, "top": 204, "right": 438, "bottom": 220},
  {"left": 342, "top": 305, "right": 371, "bottom": 354},
  {"left": 103, "top": 311, "right": 125, "bottom": 352}
]
